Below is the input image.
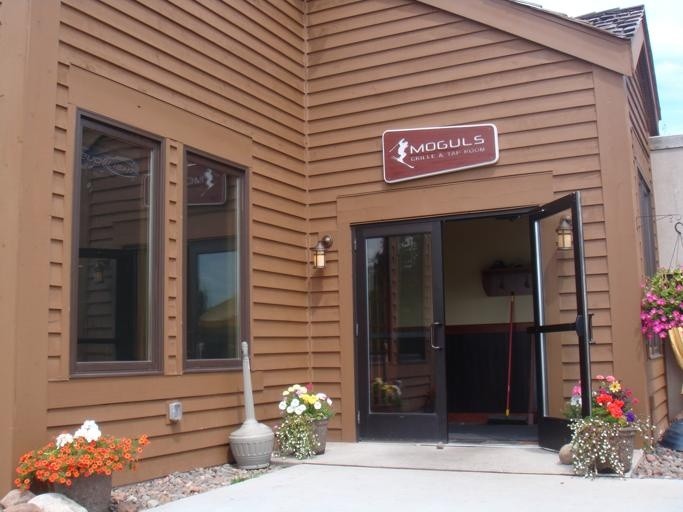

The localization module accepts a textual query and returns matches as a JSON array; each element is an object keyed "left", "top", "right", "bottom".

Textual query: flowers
[
  {"left": 560, "top": 376, "right": 660, "bottom": 482},
  {"left": 372, "top": 377, "right": 403, "bottom": 405},
  {"left": 639, "top": 267, "right": 683, "bottom": 374},
  {"left": 15, "top": 421, "right": 151, "bottom": 492},
  {"left": 274, "top": 383, "right": 333, "bottom": 461}
]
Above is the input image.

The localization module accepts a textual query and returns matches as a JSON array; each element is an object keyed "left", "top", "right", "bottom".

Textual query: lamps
[
  {"left": 554, "top": 215, "right": 573, "bottom": 250},
  {"left": 309, "top": 235, "right": 332, "bottom": 269}
]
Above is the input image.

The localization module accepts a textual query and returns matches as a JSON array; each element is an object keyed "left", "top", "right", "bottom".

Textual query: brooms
[{"left": 486, "top": 291, "right": 529, "bottom": 424}]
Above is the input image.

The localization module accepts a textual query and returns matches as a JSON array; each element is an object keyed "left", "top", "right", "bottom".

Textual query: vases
[
  {"left": 373, "top": 403, "right": 401, "bottom": 413},
  {"left": 46, "top": 473, "right": 112, "bottom": 512},
  {"left": 587, "top": 427, "right": 637, "bottom": 475},
  {"left": 668, "top": 326, "right": 683, "bottom": 373},
  {"left": 292, "top": 419, "right": 328, "bottom": 456}
]
